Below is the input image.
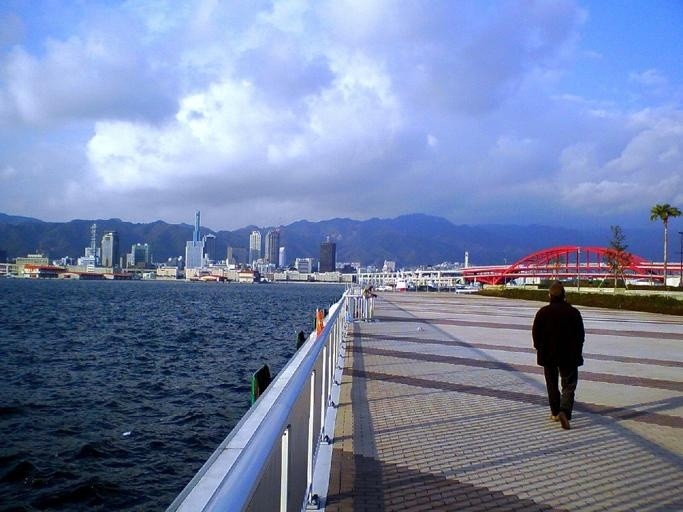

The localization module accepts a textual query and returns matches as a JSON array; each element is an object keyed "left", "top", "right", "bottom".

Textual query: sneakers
[{"left": 551, "top": 411, "right": 570, "bottom": 429}]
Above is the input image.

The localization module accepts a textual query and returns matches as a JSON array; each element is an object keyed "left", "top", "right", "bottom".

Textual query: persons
[{"left": 532, "top": 281, "right": 585, "bottom": 430}]
